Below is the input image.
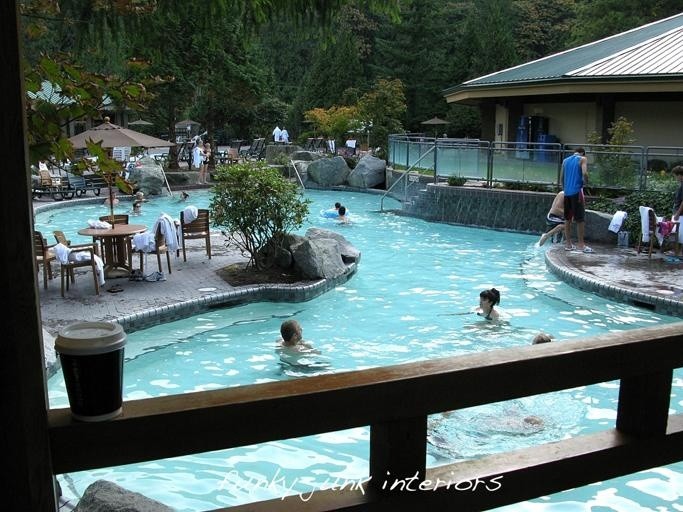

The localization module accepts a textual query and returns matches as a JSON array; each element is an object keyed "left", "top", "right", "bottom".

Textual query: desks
[{"left": 77, "top": 224, "right": 147, "bottom": 281}]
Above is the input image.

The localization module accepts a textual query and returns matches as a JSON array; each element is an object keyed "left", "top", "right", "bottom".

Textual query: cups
[{"left": 54, "top": 321, "right": 128, "bottom": 422}]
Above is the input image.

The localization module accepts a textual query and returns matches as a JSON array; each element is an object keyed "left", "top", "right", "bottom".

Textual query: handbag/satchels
[{"left": 618, "top": 231, "right": 628, "bottom": 246}]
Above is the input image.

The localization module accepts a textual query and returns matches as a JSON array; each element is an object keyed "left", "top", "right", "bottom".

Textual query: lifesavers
[{"left": 323, "top": 207, "right": 347, "bottom": 218}]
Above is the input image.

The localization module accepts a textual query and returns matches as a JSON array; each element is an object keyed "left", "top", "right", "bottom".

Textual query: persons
[
  {"left": 336, "top": 207, "right": 348, "bottom": 220},
  {"left": 105, "top": 125, "right": 289, "bottom": 216},
  {"left": 480, "top": 289, "right": 500, "bottom": 318},
  {"left": 330, "top": 203, "right": 348, "bottom": 212},
  {"left": 281, "top": 320, "right": 306, "bottom": 351},
  {"left": 533, "top": 335, "right": 551, "bottom": 345},
  {"left": 666, "top": 166, "right": 683, "bottom": 256},
  {"left": 540, "top": 191, "right": 573, "bottom": 246},
  {"left": 560, "top": 148, "right": 596, "bottom": 253}
]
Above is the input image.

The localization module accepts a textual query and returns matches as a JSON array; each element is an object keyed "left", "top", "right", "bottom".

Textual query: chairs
[
  {"left": 636, "top": 205, "right": 680, "bottom": 260},
  {"left": 174, "top": 208, "right": 212, "bottom": 262},
  {"left": 127, "top": 218, "right": 172, "bottom": 275},
  {"left": 100, "top": 215, "right": 129, "bottom": 270},
  {"left": 52, "top": 231, "right": 102, "bottom": 297},
  {"left": 34, "top": 231, "right": 70, "bottom": 289}
]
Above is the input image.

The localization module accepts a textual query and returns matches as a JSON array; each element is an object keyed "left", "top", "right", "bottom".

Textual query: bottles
[{"left": 224, "top": 149, "right": 228, "bottom": 164}]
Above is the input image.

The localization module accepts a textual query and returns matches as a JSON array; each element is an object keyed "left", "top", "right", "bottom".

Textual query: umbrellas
[
  {"left": 68, "top": 116, "right": 176, "bottom": 270},
  {"left": 420, "top": 116, "right": 451, "bottom": 137},
  {"left": 128, "top": 118, "right": 154, "bottom": 124},
  {"left": 177, "top": 119, "right": 200, "bottom": 125}
]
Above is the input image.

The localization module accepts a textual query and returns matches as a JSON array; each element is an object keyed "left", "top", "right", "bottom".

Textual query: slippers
[{"left": 106, "top": 284, "right": 123, "bottom": 293}]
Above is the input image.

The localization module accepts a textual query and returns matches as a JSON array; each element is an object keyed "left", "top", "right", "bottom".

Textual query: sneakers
[
  {"left": 144, "top": 271, "right": 167, "bottom": 282},
  {"left": 666, "top": 256, "right": 683, "bottom": 263},
  {"left": 564, "top": 244, "right": 596, "bottom": 253},
  {"left": 641, "top": 247, "right": 655, "bottom": 253},
  {"left": 129, "top": 269, "right": 143, "bottom": 281}
]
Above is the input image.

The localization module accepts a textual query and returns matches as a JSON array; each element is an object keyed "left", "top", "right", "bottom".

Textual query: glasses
[{"left": 135, "top": 206, "right": 141, "bottom": 208}]
